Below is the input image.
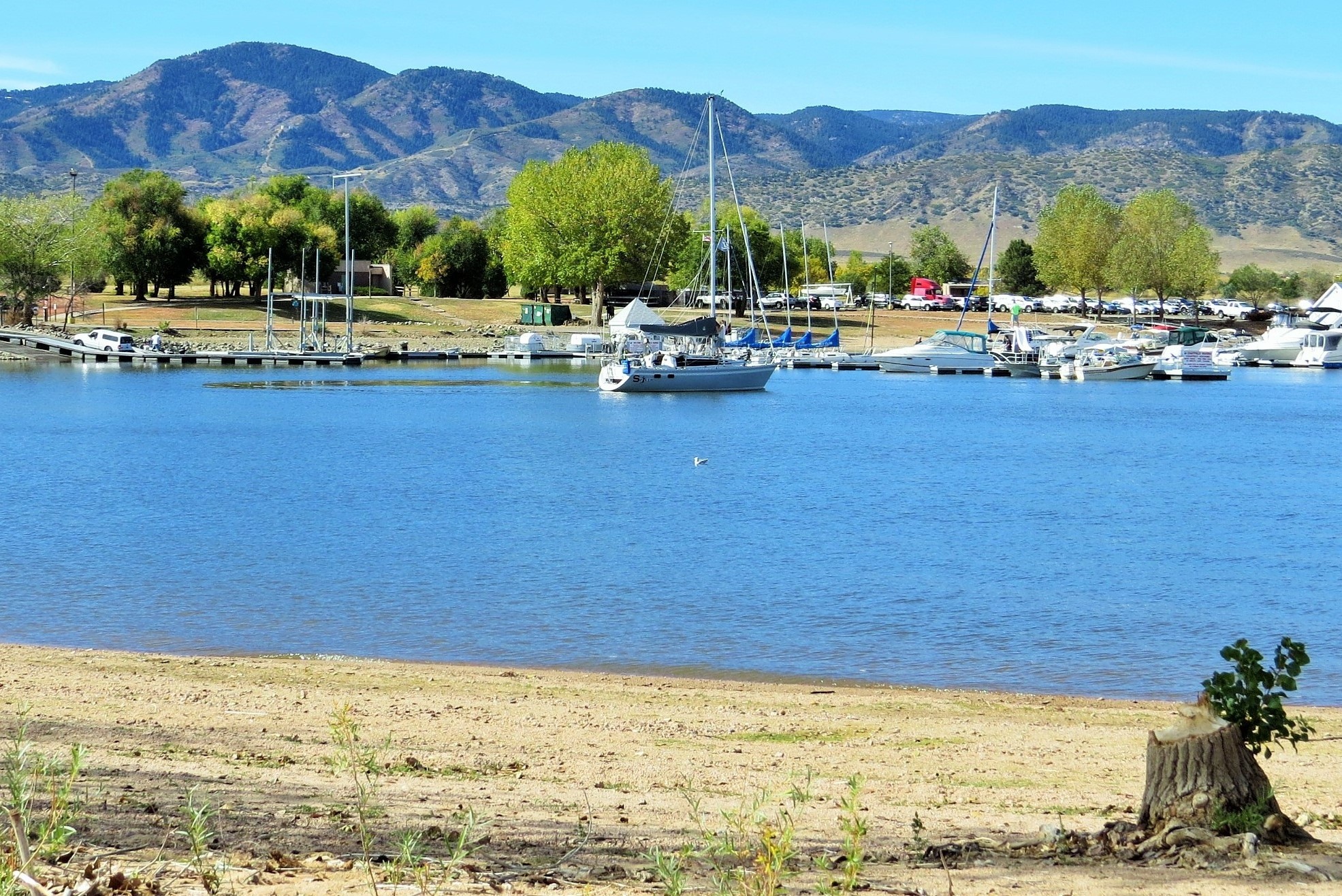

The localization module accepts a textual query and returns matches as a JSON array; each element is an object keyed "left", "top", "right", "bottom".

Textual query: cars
[
  {"left": 1111, "top": 296, "right": 1228, "bottom": 317},
  {"left": 1038, "top": 298, "right": 1070, "bottom": 313},
  {"left": 963, "top": 295, "right": 995, "bottom": 312},
  {"left": 1096, "top": 300, "right": 1118, "bottom": 315},
  {"left": 820, "top": 297, "right": 844, "bottom": 311},
  {"left": 794, "top": 295, "right": 822, "bottom": 310},
  {"left": 9, "top": 297, "right": 39, "bottom": 317},
  {"left": 0, "top": 296, "right": 12, "bottom": 312},
  {"left": 1078, "top": 298, "right": 1103, "bottom": 313}
]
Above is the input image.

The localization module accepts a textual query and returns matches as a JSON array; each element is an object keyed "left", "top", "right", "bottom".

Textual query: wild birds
[{"left": 694, "top": 457, "right": 708, "bottom": 467}]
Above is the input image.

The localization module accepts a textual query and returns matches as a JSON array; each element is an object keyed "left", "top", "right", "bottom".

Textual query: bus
[{"left": 941, "top": 282, "right": 995, "bottom": 312}]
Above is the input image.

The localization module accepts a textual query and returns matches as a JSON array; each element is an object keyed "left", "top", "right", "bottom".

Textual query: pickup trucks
[{"left": 1214, "top": 301, "right": 1259, "bottom": 320}]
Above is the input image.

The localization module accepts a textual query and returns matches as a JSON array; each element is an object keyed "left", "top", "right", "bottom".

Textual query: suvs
[
  {"left": 696, "top": 291, "right": 743, "bottom": 309},
  {"left": 1063, "top": 297, "right": 1087, "bottom": 314},
  {"left": 756, "top": 293, "right": 796, "bottom": 309},
  {"left": 900, "top": 294, "right": 940, "bottom": 310}
]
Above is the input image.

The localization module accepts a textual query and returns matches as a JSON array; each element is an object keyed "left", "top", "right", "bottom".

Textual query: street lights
[{"left": 69, "top": 168, "right": 76, "bottom": 324}]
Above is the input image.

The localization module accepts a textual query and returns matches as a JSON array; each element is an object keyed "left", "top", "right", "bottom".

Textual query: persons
[
  {"left": 151, "top": 332, "right": 161, "bottom": 351},
  {"left": 1009, "top": 297, "right": 1022, "bottom": 327},
  {"left": 606, "top": 303, "right": 614, "bottom": 321},
  {"left": 915, "top": 337, "right": 923, "bottom": 345},
  {"left": 1001, "top": 336, "right": 1012, "bottom": 351}
]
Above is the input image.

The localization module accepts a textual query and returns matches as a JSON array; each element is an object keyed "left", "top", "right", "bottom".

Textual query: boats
[{"left": 872, "top": 306, "right": 1342, "bottom": 380}]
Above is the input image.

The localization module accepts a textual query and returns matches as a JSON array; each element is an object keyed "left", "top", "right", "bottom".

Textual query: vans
[
  {"left": 73, "top": 329, "right": 133, "bottom": 352},
  {"left": 999, "top": 296, "right": 1043, "bottom": 313}
]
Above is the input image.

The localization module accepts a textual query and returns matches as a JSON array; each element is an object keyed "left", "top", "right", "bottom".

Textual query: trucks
[
  {"left": 890, "top": 277, "right": 952, "bottom": 311},
  {"left": 589, "top": 281, "right": 660, "bottom": 307},
  {"left": 853, "top": 292, "right": 894, "bottom": 308}
]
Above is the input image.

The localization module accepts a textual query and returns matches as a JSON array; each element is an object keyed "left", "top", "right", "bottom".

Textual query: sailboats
[
  {"left": 598, "top": 95, "right": 779, "bottom": 392},
  {"left": 693, "top": 219, "right": 881, "bottom": 368}
]
